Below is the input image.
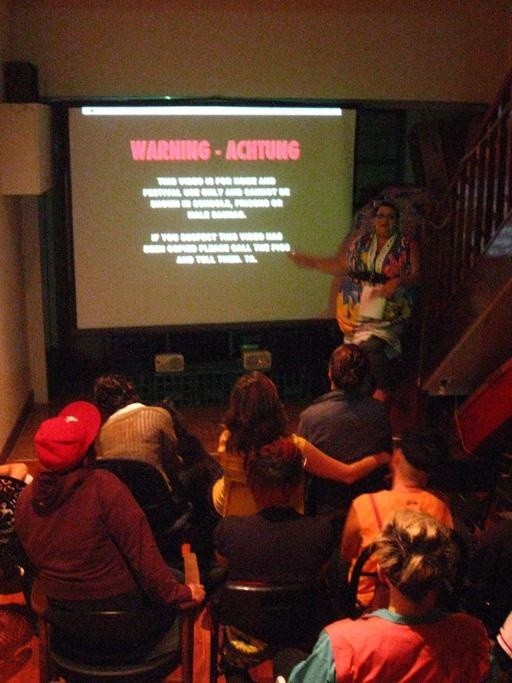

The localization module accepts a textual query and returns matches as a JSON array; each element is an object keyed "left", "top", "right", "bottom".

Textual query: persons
[
  {"left": 1, "top": 343, "right": 512, "bottom": 683},
  {"left": 288, "top": 200, "right": 426, "bottom": 362}
]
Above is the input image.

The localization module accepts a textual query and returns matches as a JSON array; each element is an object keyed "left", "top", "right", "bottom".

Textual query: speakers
[
  {"left": 154, "top": 351, "right": 186, "bottom": 373},
  {"left": 240, "top": 348, "right": 273, "bottom": 370}
]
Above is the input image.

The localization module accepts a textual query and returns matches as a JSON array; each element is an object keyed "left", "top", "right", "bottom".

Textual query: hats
[{"left": 33, "top": 399, "right": 101, "bottom": 468}]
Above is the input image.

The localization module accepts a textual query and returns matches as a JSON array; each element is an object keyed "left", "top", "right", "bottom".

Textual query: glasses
[
  {"left": 299, "top": 455, "right": 307, "bottom": 470},
  {"left": 375, "top": 214, "right": 394, "bottom": 219}
]
